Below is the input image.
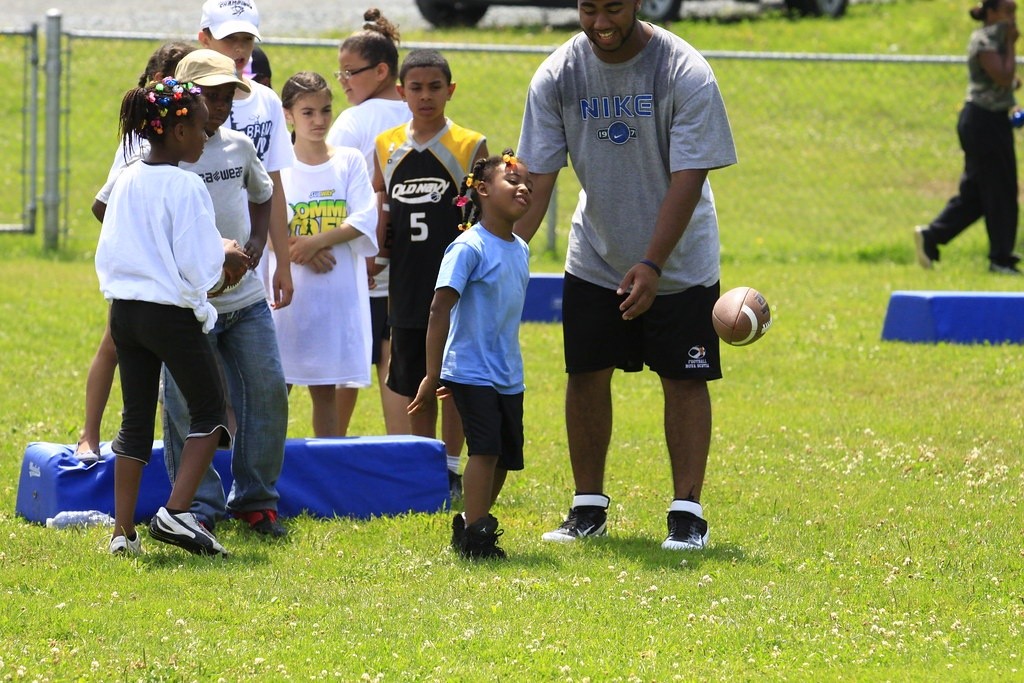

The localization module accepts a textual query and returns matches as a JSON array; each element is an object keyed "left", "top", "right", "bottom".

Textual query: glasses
[{"left": 335, "top": 64, "right": 380, "bottom": 80}]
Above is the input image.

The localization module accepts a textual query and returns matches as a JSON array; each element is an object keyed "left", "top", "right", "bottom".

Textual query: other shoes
[
  {"left": 988, "top": 263, "right": 1024, "bottom": 276},
  {"left": 915, "top": 225, "right": 943, "bottom": 271},
  {"left": 72, "top": 441, "right": 102, "bottom": 463},
  {"left": 448, "top": 469, "right": 463, "bottom": 502},
  {"left": 452, "top": 512, "right": 509, "bottom": 561},
  {"left": 227, "top": 505, "right": 287, "bottom": 537}
]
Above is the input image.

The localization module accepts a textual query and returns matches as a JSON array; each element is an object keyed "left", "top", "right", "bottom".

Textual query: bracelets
[{"left": 639, "top": 257, "right": 663, "bottom": 278}]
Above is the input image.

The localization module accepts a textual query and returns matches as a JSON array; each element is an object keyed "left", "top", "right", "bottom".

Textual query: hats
[
  {"left": 200, "top": 0, "right": 263, "bottom": 40},
  {"left": 181, "top": 47, "right": 251, "bottom": 95},
  {"left": 247, "top": 45, "right": 272, "bottom": 79}
]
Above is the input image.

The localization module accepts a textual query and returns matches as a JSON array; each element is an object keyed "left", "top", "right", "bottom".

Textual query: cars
[{"left": 412, "top": 0, "right": 850, "bottom": 32}]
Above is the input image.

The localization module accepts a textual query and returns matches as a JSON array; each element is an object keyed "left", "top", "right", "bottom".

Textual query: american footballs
[
  {"left": 206, "top": 237, "right": 241, "bottom": 299},
  {"left": 711, "top": 286, "right": 772, "bottom": 348}
]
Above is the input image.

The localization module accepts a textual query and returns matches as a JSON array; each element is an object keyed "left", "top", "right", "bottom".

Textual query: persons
[
  {"left": 72, "top": 41, "right": 199, "bottom": 460},
  {"left": 328, "top": 8, "right": 413, "bottom": 434},
  {"left": 512, "top": 0, "right": 737, "bottom": 557},
  {"left": 92, "top": 75, "right": 249, "bottom": 559},
  {"left": 408, "top": 147, "right": 532, "bottom": 561},
  {"left": 365, "top": 45, "right": 490, "bottom": 491},
  {"left": 914, "top": 0, "right": 1024, "bottom": 274},
  {"left": 273, "top": 70, "right": 381, "bottom": 437},
  {"left": 198, "top": 0, "right": 294, "bottom": 311},
  {"left": 92, "top": 49, "right": 295, "bottom": 539}
]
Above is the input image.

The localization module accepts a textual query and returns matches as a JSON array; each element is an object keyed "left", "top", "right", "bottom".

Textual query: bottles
[{"left": 46, "top": 510, "right": 104, "bottom": 531}]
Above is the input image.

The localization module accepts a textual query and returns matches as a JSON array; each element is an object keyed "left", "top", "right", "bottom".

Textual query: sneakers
[
  {"left": 541, "top": 491, "right": 610, "bottom": 546},
  {"left": 148, "top": 505, "right": 229, "bottom": 560},
  {"left": 109, "top": 529, "right": 145, "bottom": 558},
  {"left": 659, "top": 499, "right": 709, "bottom": 552}
]
